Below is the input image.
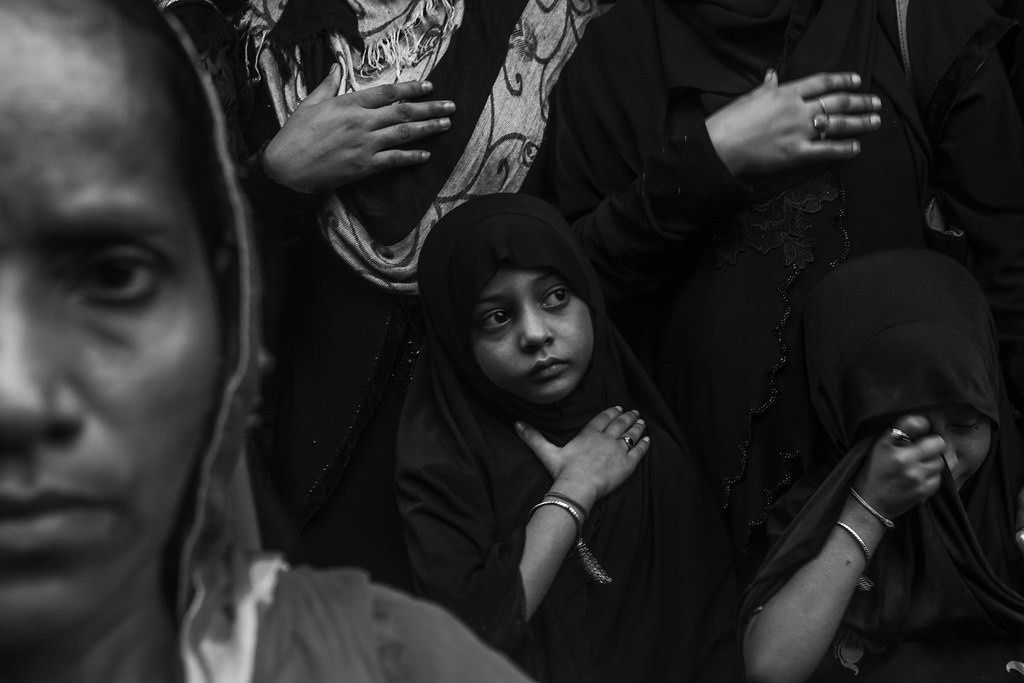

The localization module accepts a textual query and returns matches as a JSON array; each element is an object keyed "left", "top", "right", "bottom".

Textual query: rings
[
  {"left": 814, "top": 114, "right": 831, "bottom": 142},
  {"left": 817, "top": 97, "right": 830, "bottom": 115},
  {"left": 886, "top": 425, "right": 913, "bottom": 446},
  {"left": 617, "top": 433, "right": 634, "bottom": 451}
]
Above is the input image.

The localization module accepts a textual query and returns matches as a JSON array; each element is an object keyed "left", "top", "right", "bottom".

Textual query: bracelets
[
  {"left": 850, "top": 487, "right": 897, "bottom": 531},
  {"left": 832, "top": 520, "right": 871, "bottom": 566},
  {"left": 543, "top": 491, "right": 590, "bottom": 524},
  {"left": 529, "top": 501, "right": 612, "bottom": 587}
]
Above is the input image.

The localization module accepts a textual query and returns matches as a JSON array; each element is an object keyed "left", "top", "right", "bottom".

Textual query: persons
[{"left": 0, "top": 0, "right": 1024, "bottom": 683}]
